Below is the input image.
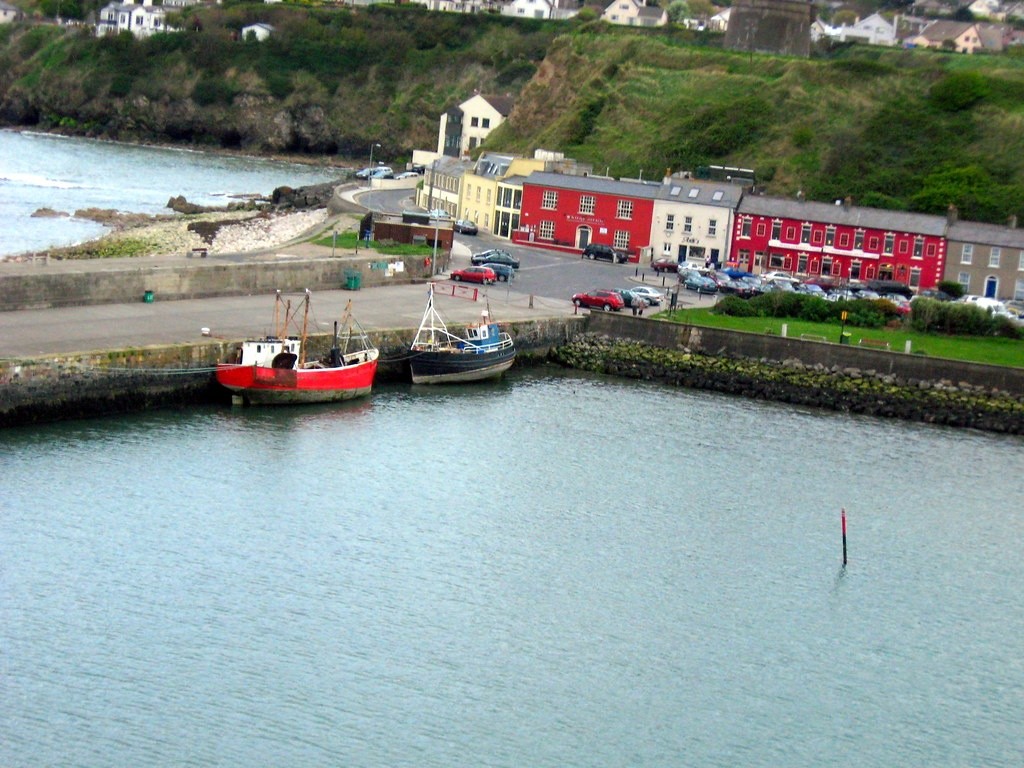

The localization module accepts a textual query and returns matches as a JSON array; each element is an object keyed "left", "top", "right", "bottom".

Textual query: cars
[
  {"left": 356, "top": 166, "right": 425, "bottom": 180},
  {"left": 452, "top": 220, "right": 478, "bottom": 236},
  {"left": 684, "top": 276, "right": 718, "bottom": 296},
  {"left": 719, "top": 267, "right": 1024, "bottom": 321},
  {"left": 583, "top": 243, "right": 628, "bottom": 265},
  {"left": 471, "top": 253, "right": 521, "bottom": 269},
  {"left": 472, "top": 249, "right": 513, "bottom": 257},
  {"left": 706, "top": 271, "right": 731, "bottom": 286},
  {"left": 677, "top": 261, "right": 711, "bottom": 276},
  {"left": 572, "top": 290, "right": 625, "bottom": 312},
  {"left": 630, "top": 286, "right": 665, "bottom": 306},
  {"left": 451, "top": 266, "right": 497, "bottom": 284},
  {"left": 679, "top": 269, "right": 701, "bottom": 283},
  {"left": 652, "top": 258, "right": 680, "bottom": 274},
  {"left": 432, "top": 209, "right": 451, "bottom": 219},
  {"left": 480, "top": 263, "right": 515, "bottom": 282},
  {"left": 614, "top": 288, "right": 650, "bottom": 309}
]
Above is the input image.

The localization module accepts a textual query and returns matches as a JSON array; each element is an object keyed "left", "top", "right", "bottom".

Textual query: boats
[
  {"left": 406, "top": 282, "right": 517, "bottom": 386},
  {"left": 217, "top": 288, "right": 379, "bottom": 407}
]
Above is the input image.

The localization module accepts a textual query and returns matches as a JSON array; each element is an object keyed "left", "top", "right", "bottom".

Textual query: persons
[
  {"left": 632, "top": 296, "right": 645, "bottom": 315},
  {"left": 705, "top": 256, "right": 711, "bottom": 268}
]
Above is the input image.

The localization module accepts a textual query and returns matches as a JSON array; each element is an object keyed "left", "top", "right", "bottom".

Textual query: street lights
[{"left": 368, "top": 144, "right": 385, "bottom": 210}]
